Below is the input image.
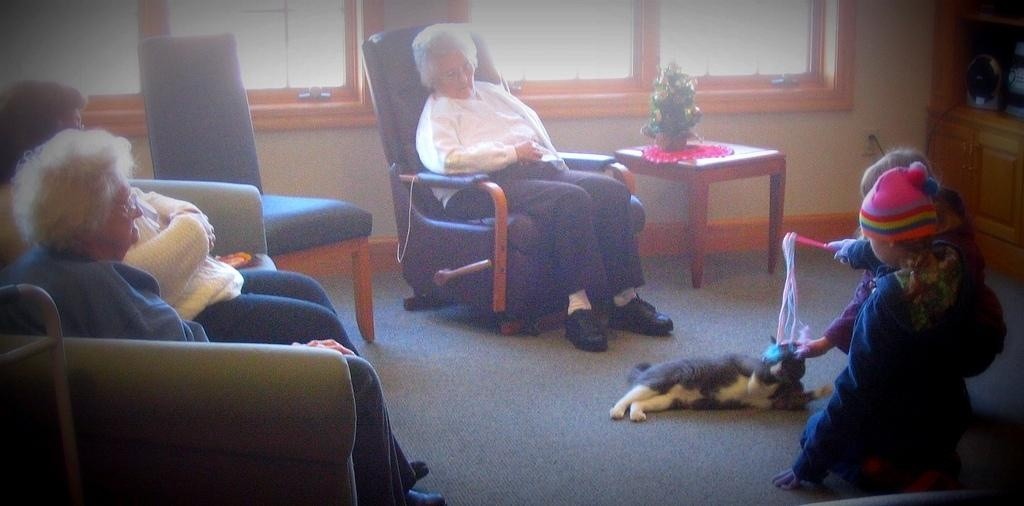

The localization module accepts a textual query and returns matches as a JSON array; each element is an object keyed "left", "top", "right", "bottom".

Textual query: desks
[{"left": 613, "top": 136, "right": 787, "bottom": 291}]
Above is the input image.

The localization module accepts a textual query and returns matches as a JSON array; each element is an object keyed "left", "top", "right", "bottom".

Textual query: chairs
[
  {"left": 359, "top": 22, "right": 647, "bottom": 337},
  {"left": 136, "top": 32, "right": 377, "bottom": 344}
]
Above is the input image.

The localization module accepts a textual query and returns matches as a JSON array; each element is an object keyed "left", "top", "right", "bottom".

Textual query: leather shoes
[
  {"left": 608, "top": 295, "right": 673, "bottom": 336},
  {"left": 566, "top": 309, "right": 607, "bottom": 351},
  {"left": 404, "top": 462, "right": 445, "bottom": 506}
]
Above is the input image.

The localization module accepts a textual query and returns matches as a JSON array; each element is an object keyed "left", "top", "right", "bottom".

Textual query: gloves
[
  {"left": 828, "top": 239, "right": 856, "bottom": 264},
  {"left": 772, "top": 468, "right": 799, "bottom": 490}
]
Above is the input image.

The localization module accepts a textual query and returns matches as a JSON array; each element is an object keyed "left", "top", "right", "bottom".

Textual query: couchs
[{"left": 0, "top": 178, "right": 364, "bottom": 506}]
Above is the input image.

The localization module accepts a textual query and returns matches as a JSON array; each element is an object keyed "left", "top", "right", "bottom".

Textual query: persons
[
  {"left": 795, "top": 149, "right": 984, "bottom": 360},
  {"left": 774, "top": 162, "right": 982, "bottom": 493},
  {"left": 0, "top": 79, "right": 364, "bottom": 360},
  {"left": 411, "top": 22, "right": 674, "bottom": 350},
  {"left": 0, "top": 128, "right": 447, "bottom": 506}
]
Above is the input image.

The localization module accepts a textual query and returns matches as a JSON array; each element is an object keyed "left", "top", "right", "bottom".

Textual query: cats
[{"left": 609, "top": 333, "right": 835, "bottom": 424}]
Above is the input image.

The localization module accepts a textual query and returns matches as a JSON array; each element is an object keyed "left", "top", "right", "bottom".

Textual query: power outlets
[{"left": 860, "top": 128, "right": 879, "bottom": 157}]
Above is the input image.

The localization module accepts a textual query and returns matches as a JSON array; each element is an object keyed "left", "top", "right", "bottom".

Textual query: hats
[{"left": 859, "top": 149, "right": 939, "bottom": 241}]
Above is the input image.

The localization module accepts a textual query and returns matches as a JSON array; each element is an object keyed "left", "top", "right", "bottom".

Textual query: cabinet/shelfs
[{"left": 921, "top": 119, "right": 1022, "bottom": 283}]
